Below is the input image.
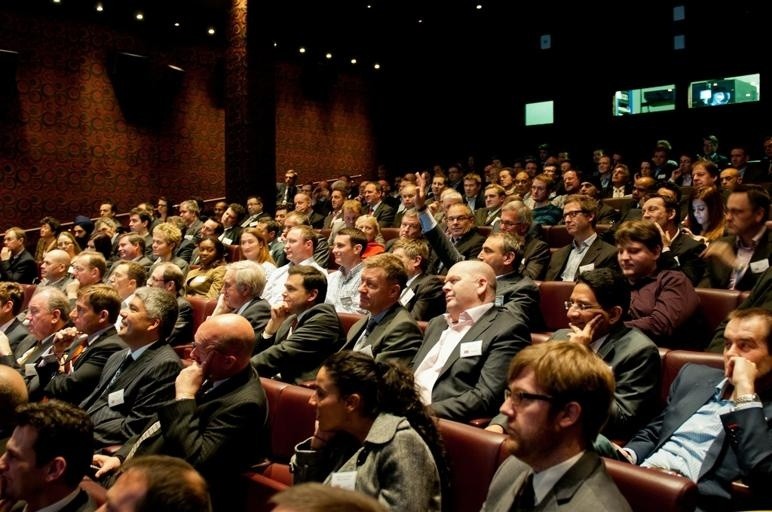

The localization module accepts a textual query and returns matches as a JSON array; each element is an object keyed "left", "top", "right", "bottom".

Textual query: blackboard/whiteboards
[{"left": 525, "top": 101, "right": 554, "bottom": 126}]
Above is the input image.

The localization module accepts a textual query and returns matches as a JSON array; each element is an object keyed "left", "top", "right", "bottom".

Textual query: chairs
[
  {"left": 437, "top": 275, "right": 447, "bottom": 282},
  {"left": 259, "top": 375, "right": 290, "bottom": 427},
  {"left": 336, "top": 312, "right": 364, "bottom": 337},
  {"left": 394, "top": 228, "right": 400, "bottom": 239},
  {"left": 203, "top": 300, "right": 218, "bottom": 322},
  {"left": 79, "top": 478, "right": 107, "bottom": 509},
  {"left": 327, "top": 269, "right": 336, "bottom": 274},
  {"left": 23, "top": 284, "right": 35, "bottom": 310},
  {"left": 417, "top": 321, "right": 427, "bottom": 331},
  {"left": 739, "top": 291, "right": 750, "bottom": 302},
  {"left": 228, "top": 245, "right": 240, "bottom": 264},
  {"left": 539, "top": 280, "right": 574, "bottom": 331},
  {"left": 552, "top": 224, "right": 611, "bottom": 249},
  {"left": 180, "top": 357, "right": 194, "bottom": 369},
  {"left": 247, "top": 473, "right": 284, "bottom": 508},
  {"left": 533, "top": 280, "right": 541, "bottom": 287},
  {"left": 497, "top": 439, "right": 693, "bottom": 511},
  {"left": 693, "top": 288, "right": 740, "bottom": 330},
  {"left": 477, "top": 226, "right": 491, "bottom": 236},
  {"left": 748, "top": 160, "right": 761, "bottom": 175},
  {"left": 658, "top": 347, "right": 670, "bottom": 362},
  {"left": 272, "top": 386, "right": 317, "bottom": 463},
  {"left": 530, "top": 332, "right": 548, "bottom": 345},
  {"left": 380, "top": 228, "right": 395, "bottom": 241},
  {"left": 190, "top": 265, "right": 199, "bottom": 269},
  {"left": 327, "top": 246, "right": 341, "bottom": 268},
  {"left": 425, "top": 415, "right": 510, "bottom": 512},
  {"left": 319, "top": 229, "right": 331, "bottom": 241},
  {"left": 602, "top": 198, "right": 633, "bottom": 210},
  {"left": 20, "top": 283, "right": 32, "bottom": 310},
  {"left": 184, "top": 297, "right": 209, "bottom": 340},
  {"left": 662, "top": 350, "right": 725, "bottom": 406},
  {"left": 541, "top": 225, "right": 551, "bottom": 243}
]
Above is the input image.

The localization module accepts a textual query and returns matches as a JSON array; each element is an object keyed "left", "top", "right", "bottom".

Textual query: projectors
[{"left": 639, "top": 87, "right": 676, "bottom": 108}]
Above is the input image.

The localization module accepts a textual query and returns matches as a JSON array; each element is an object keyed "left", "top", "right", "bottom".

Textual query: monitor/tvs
[{"left": 687, "top": 73, "right": 739, "bottom": 109}]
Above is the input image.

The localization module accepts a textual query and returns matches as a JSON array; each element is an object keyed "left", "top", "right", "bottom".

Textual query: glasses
[
  {"left": 246, "top": 203, "right": 261, "bottom": 207},
  {"left": 152, "top": 277, "right": 175, "bottom": 284},
  {"left": 503, "top": 387, "right": 574, "bottom": 407},
  {"left": 563, "top": 210, "right": 587, "bottom": 219},
  {"left": 565, "top": 301, "right": 608, "bottom": 312},
  {"left": 447, "top": 216, "right": 473, "bottom": 222},
  {"left": 500, "top": 221, "right": 524, "bottom": 228},
  {"left": 72, "top": 265, "right": 95, "bottom": 273}
]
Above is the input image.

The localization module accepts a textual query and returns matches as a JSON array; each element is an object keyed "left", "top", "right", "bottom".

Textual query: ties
[
  {"left": 88, "top": 354, "right": 133, "bottom": 411},
  {"left": 351, "top": 319, "right": 376, "bottom": 354},
  {"left": 240, "top": 216, "right": 254, "bottom": 226},
  {"left": 513, "top": 475, "right": 531, "bottom": 512},
  {"left": 36, "top": 338, "right": 88, "bottom": 404},
  {"left": 17, "top": 340, "right": 44, "bottom": 366},
  {"left": 278, "top": 316, "right": 297, "bottom": 343},
  {"left": 122, "top": 381, "right": 215, "bottom": 467}
]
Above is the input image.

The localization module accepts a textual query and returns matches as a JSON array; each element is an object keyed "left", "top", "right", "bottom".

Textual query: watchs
[
  {"left": 735, "top": 392, "right": 759, "bottom": 402},
  {"left": 417, "top": 205, "right": 428, "bottom": 213},
  {"left": 263, "top": 327, "right": 277, "bottom": 334}
]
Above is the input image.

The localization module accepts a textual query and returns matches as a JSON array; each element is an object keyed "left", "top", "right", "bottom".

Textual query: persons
[
  {"left": 99, "top": 458, "right": 213, "bottom": 511},
  {"left": 426, "top": 206, "right": 486, "bottom": 277},
  {"left": 413, "top": 154, "right": 539, "bottom": 202},
  {"left": 622, "top": 309, "right": 771, "bottom": 510},
  {"left": 288, "top": 351, "right": 447, "bottom": 511},
  {"left": 33, "top": 195, "right": 187, "bottom": 308},
  {"left": 262, "top": 226, "right": 327, "bottom": 309},
  {"left": 488, "top": 265, "right": 661, "bottom": 459},
  {"left": 351, "top": 164, "right": 430, "bottom": 259},
  {"left": 11, "top": 289, "right": 72, "bottom": 374},
  {"left": 0, "top": 229, "right": 34, "bottom": 280},
  {"left": 544, "top": 196, "right": 628, "bottom": 302},
  {"left": 180, "top": 192, "right": 278, "bottom": 267},
  {"left": 89, "top": 313, "right": 268, "bottom": 505},
  {"left": 613, "top": 224, "right": 704, "bottom": 345},
  {"left": 493, "top": 201, "right": 550, "bottom": 278},
  {"left": 632, "top": 196, "right": 708, "bottom": 284},
  {"left": 296, "top": 254, "right": 425, "bottom": 387},
  {"left": 72, "top": 285, "right": 182, "bottom": 450},
  {"left": 321, "top": 229, "right": 369, "bottom": 314},
  {"left": 705, "top": 186, "right": 772, "bottom": 294},
  {"left": 409, "top": 260, "right": 527, "bottom": 425},
  {"left": 530, "top": 135, "right": 772, "bottom": 215},
  {"left": 247, "top": 265, "right": 345, "bottom": 386},
  {"left": 0, "top": 282, "right": 28, "bottom": 350},
  {"left": 211, "top": 261, "right": 271, "bottom": 342},
  {"left": 1, "top": 401, "right": 97, "bottom": 512},
  {"left": 187, "top": 239, "right": 225, "bottom": 312},
  {"left": 273, "top": 166, "right": 363, "bottom": 237},
  {"left": 35, "top": 285, "right": 125, "bottom": 401},
  {"left": 477, "top": 344, "right": 629, "bottom": 511}
]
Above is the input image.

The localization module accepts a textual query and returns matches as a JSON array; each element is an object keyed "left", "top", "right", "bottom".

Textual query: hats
[
  {"left": 74, "top": 216, "right": 95, "bottom": 234},
  {"left": 579, "top": 175, "right": 602, "bottom": 190}
]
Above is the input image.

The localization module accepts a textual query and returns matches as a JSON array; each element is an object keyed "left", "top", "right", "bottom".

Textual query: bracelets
[{"left": 312, "top": 433, "right": 324, "bottom": 440}]
